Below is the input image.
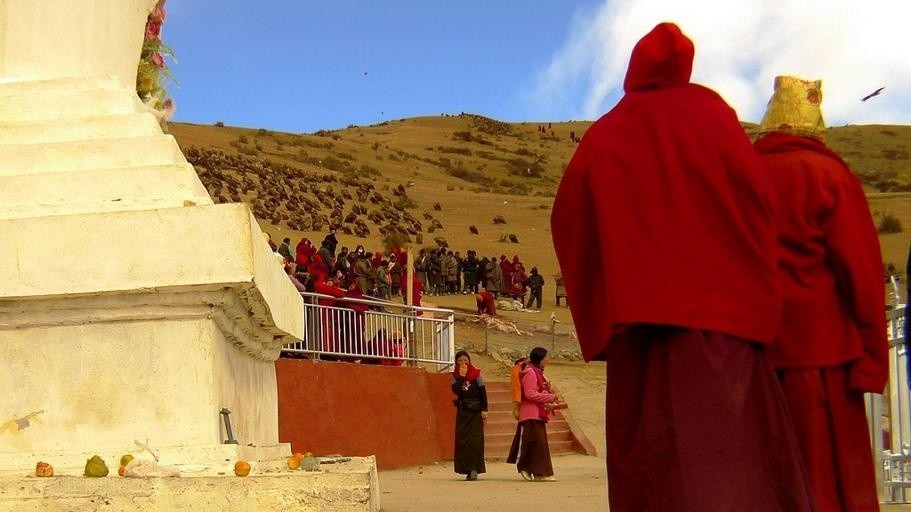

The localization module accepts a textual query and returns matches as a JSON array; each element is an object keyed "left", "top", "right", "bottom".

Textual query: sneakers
[
  {"left": 520, "top": 470, "right": 532, "bottom": 481},
  {"left": 534, "top": 475, "right": 557, "bottom": 482}
]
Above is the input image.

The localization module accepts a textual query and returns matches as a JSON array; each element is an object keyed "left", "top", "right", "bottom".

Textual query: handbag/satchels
[{"left": 462, "top": 398, "right": 482, "bottom": 412}]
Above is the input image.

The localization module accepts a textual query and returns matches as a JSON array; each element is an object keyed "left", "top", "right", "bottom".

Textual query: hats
[{"left": 758, "top": 76, "right": 826, "bottom": 139}]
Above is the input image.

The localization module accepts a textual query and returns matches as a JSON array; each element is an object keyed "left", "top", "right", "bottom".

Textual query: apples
[
  {"left": 118, "top": 467, "right": 125, "bottom": 475},
  {"left": 120, "top": 454, "right": 134, "bottom": 464},
  {"left": 232, "top": 460, "right": 251, "bottom": 477},
  {"left": 287, "top": 451, "right": 312, "bottom": 469}
]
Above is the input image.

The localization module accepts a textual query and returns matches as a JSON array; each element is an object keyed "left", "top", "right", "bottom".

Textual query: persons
[
  {"left": 262, "top": 225, "right": 545, "bottom": 366},
  {"left": 509, "top": 358, "right": 527, "bottom": 420},
  {"left": 513, "top": 347, "right": 561, "bottom": 482},
  {"left": 755, "top": 76, "right": 890, "bottom": 511},
  {"left": 451, "top": 351, "right": 489, "bottom": 481},
  {"left": 549, "top": 22, "right": 788, "bottom": 512}
]
